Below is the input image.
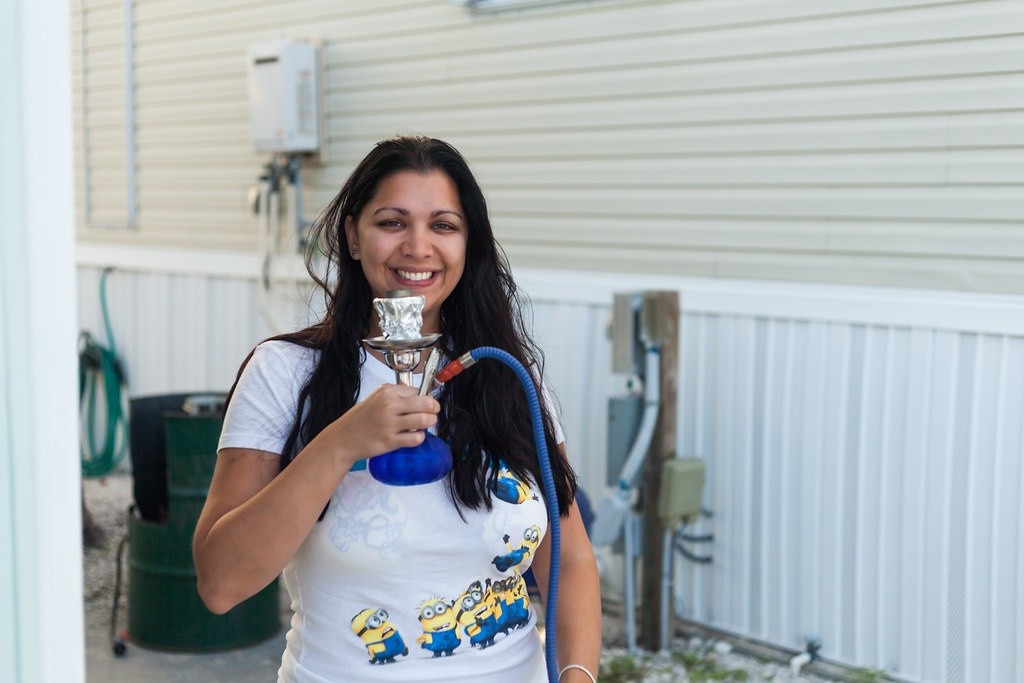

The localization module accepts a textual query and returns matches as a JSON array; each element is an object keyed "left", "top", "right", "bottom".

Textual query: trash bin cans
[{"left": 123, "top": 390, "right": 284, "bottom": 657}]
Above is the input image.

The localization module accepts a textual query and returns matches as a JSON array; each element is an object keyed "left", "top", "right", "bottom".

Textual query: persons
[{"left": 193, "top": 135, "right": 601, "bottom": 683}]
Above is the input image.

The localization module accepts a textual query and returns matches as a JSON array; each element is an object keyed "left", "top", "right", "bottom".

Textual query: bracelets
[{"left": 558, "top": 664, "right": 597, "bottom": 683}]
